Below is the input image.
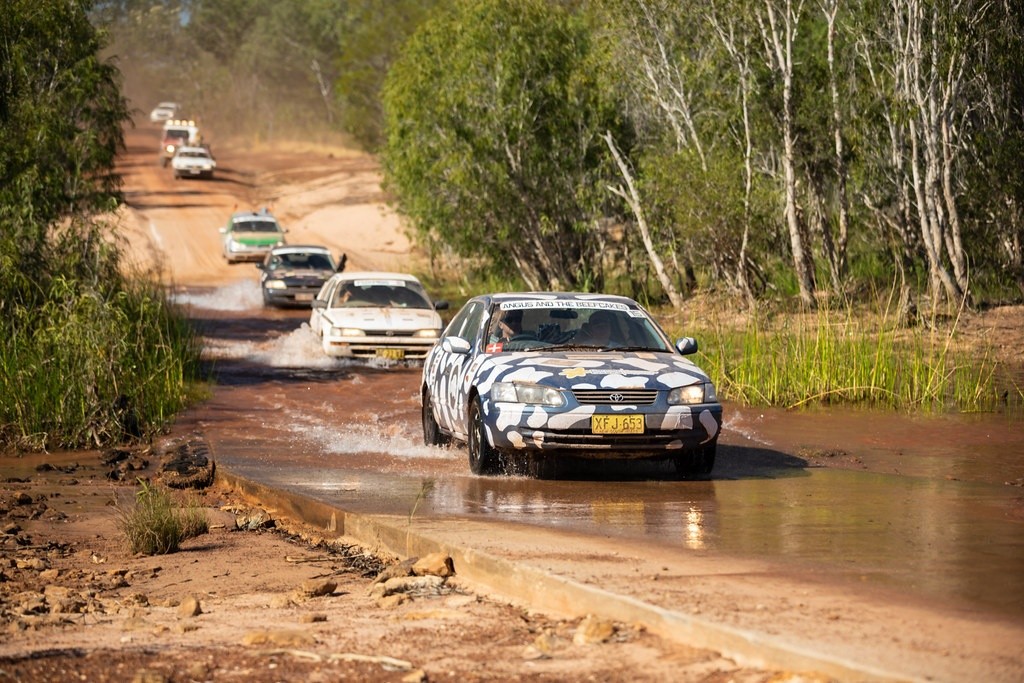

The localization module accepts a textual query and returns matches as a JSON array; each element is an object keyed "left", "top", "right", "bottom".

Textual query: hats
[{"left": 588, "top": 311, "right": 613, "bottom": 328}]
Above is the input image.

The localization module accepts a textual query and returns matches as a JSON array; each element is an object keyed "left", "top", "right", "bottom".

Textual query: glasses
[{"left": 500, "top": 316, "right": 523, "bottom": 325}]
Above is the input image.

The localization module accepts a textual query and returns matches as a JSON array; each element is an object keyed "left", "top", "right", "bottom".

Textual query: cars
[
  {"left": 170, "top": 146, "right": 217, "bottom": 180},
  {"left": 218, "top": 209, "right": 290, "bottom": 266},
  {"left": 151, "top": 101, "right": 182, "bottom": 124},
  {"left": 256, "top": 245, "right": 341, "bottom": 309},
  {"left": 309, "top": 273, "right": 451, "bottom": 367},
  {"left": 417, "top": 291, "right": 724, "bottom": 479}
]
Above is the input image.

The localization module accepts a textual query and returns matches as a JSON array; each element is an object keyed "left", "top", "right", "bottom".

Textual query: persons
[
  {"left": 575, "top": 311, "right": 628, "bottom": 350},
  {"left": 308, "top": 254, "right": 347, "bottom": 272},
  {"left": 495, "top": 310, "right": 538, "bottom": 342},
  {"left": 385, "top": 287, "right": 424, "bottom": 308},
  {"left": 276, "top": 254, "right": 291, "bottom": 267},
  {"left": 339, "top": 285, "right": 366, "bottom": 303}
]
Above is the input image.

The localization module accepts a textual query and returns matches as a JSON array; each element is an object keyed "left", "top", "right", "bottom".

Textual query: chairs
[{"left": 570, "top": 323, "right": 619, "bottom": 346}]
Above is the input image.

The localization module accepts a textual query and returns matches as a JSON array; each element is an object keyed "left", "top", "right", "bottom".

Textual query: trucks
[{"left": 162, "top": 121, "right": 201, "bottom": 169}]
